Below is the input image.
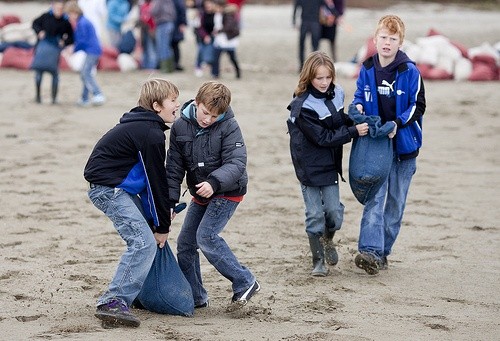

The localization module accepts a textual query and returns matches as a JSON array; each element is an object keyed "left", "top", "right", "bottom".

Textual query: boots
[{"left": 308, "top": 233, "right": 340, "bottom": 277}]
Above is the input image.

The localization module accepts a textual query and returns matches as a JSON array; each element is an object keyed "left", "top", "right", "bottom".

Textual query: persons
[
  {"left": 84, "top": 79, "right": 181, "bottom": 327},
  {"left": 353, "top": 15, "right": 426, "bottom": 275},
  {"left": 286, "top": 51, "right": 369, "bottom": 276},
  {"left": 29, "top": 3, "right": 75, "bottom": 105},
  {"left": 64, "top": 4, "right": 105, "bottom": 107},
  {"left": 106, "top": 0, "right": 242, "bottom": 79},
  {"left": 292, "top": 0, "right": 344, "bottom": 74},
  {"left": 167, "top": 80, "right": 261, "bottom": 309}
]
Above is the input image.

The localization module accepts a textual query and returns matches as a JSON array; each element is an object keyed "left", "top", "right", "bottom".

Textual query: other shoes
[
  {"left": 91, "top": 93, "right": 105, "bottom": 104},
  {"left": 354, "top": 253, "right": 388, "bottom": 275},
  {"left": 93, "top": 301, "right": 141, "bottom": 328},
  {"left": 229, "top": 280, "right": 260, "bottom": 305}
]
